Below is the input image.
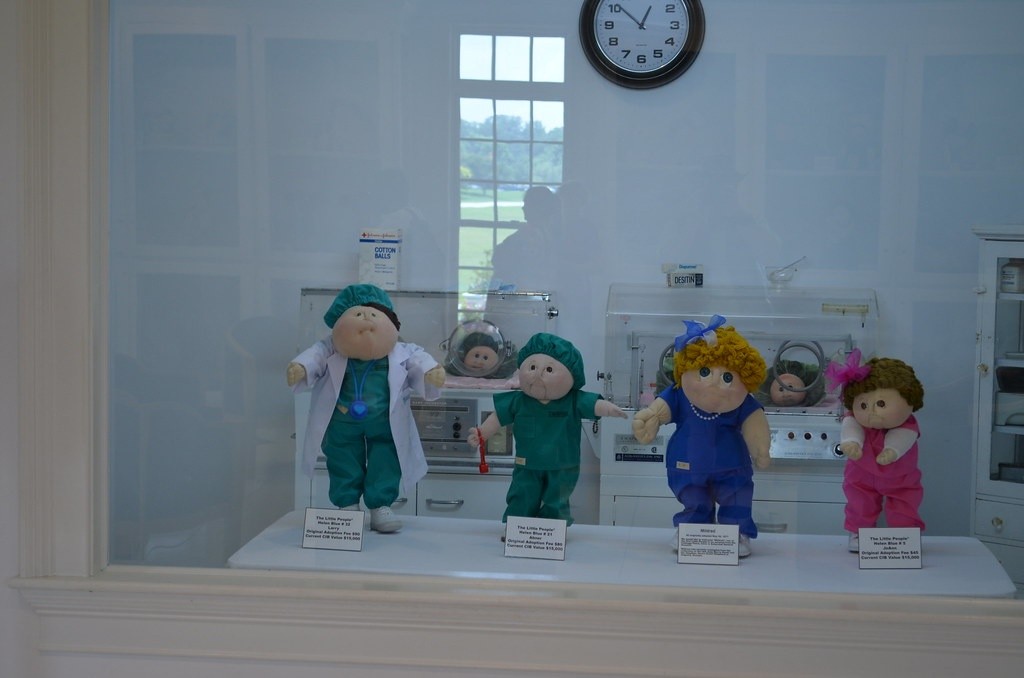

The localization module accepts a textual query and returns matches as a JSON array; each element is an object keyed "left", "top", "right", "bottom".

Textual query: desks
[{"left": 223, "top": 510, "right": 1015, "bottom": 595}]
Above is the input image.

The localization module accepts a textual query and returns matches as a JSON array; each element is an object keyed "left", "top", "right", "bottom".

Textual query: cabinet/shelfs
[
  {"left": 599, "top": 475, "right": 888, "bottom": 536},
  {"left": 309, "top": 465, "right": 543, "bottom": 521},
  {"left": 970, "top": 228, "right": 1024, "bottom": 600}
]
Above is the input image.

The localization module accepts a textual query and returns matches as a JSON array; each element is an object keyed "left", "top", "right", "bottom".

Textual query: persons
[
  {"left": 287, "top": 283, "right": 446, "bottom": 533},
  {"left": 769, "top": 374, "right": 806, "bottom": 406},
  {"left": 828, "top": 347, "right": 925, "bottom": 554},
  {"left": 467, "top": 332, "right": 629, "bottom": 542},
  {"left": 631, "top": 315, "right": 772, "bottom": 558},
  {"left": 464, "top": 346, "right": 499, "bottom": 370}
]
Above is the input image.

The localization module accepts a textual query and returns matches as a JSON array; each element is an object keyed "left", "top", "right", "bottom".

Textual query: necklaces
[{"left": 690, "top": 402, "right": 721, "bottom": 421}]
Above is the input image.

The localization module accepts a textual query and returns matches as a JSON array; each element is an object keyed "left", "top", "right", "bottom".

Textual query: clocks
[{"left": 578, "top": 0, "right": 705, "bottom": 90}]
